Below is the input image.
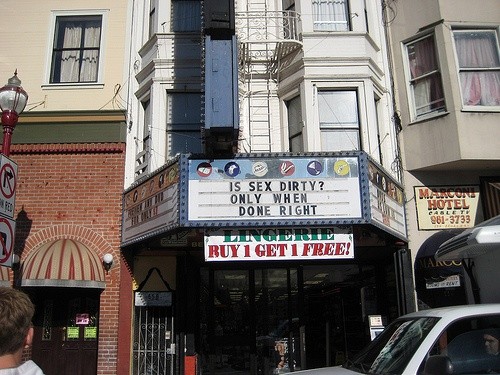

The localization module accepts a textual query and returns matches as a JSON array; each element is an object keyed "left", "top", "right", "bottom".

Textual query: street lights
[{"left": 0, "top": 69, "right": 30, "bottom": 295}]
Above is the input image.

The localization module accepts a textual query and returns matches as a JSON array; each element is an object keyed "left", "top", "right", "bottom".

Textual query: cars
[{"left": 256, "top": 318, "right": 305, "bottom": 348}]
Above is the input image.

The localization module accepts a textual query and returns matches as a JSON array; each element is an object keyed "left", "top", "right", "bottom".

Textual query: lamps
[{"left": 103, "top": 254, "right": 113, "bottom": 272}]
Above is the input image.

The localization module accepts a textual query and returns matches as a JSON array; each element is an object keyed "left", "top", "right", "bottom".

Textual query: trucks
[{"left": 281, "top": 215, "right": 500, "bottom": 374}]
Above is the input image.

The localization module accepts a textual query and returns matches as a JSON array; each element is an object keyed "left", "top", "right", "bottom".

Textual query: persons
[
  {"left": 481, "top": 325, "right": 500, "bottom": 375},
  {"left": 0, "top": 286, "right": 45, "bottom": 375}
]
